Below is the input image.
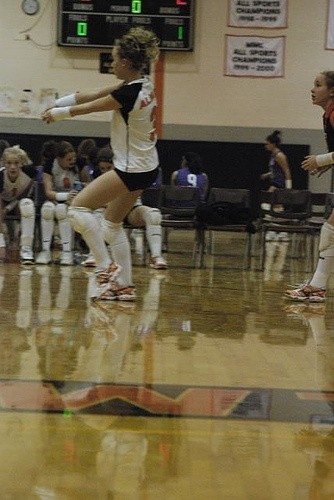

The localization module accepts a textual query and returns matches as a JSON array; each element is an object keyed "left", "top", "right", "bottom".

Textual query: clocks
[{"left": 21, "top": 0, "right": 39, "bottom": 15}]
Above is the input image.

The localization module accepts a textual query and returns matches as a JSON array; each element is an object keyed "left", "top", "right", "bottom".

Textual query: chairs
[
  {"left": 254, "top": 190, "right": 334, "bottom": 274},
  {"left": 199, "top": 188, "right": 254, "bottom": 272},
  {"left": 158, "top": 186, "right": 200, "bottom": 269},
  {"left": 123, "top": 224, "right": 148, "bottom": 267},
  {"left": 5, "top": 181, "right": 42, "bottom": 252}
]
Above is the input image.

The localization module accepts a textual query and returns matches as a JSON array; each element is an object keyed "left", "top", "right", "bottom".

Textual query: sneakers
[
  {"left": 36, "top": 251, "right": 52, "bottom": 264},
  {"left": 19, "top": 247, "right": 34, "bottom": 265},
  {"left": 135, "top": 256, "right": 145, "bottom": 266},
  {"left": 89, "top": 281, "right": 137, "bottom": 301},
  {"left": 280, "top": 303, "right": 326, "bottom": 326},
  {"left": 149, "top": 257, "right": 168, "bottom": 268},
  {"left": 84, "top": 255, "right": 97, "bottom": 267},
  {"left": 60, "top": 252, "right": 74, "bottom": 265},
  {"left": 90, "top": 301, "right": 135, "bottom": 315},
  {"left": 95, "top": 262, "right": 118, "bottom": 283},
  {"left": 283, "top": 280, "right": 326, "bottom": 302}
]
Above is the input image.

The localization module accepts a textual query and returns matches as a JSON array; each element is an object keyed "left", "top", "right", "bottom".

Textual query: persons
[
  {"left": 260, "top": 130, "right": 292, "bottom": 241},
  {"left": 0, "top": 139, "right": 167, "bottom": 270},
  {"left": 171, "top": 152, "right": 208, "bottom": 252},
  {"left": 41, "top": 27, "right": 161, "bottom": 301},
  {"left": 284, "top": 72, "right": 334, "bottom": 302}
]
